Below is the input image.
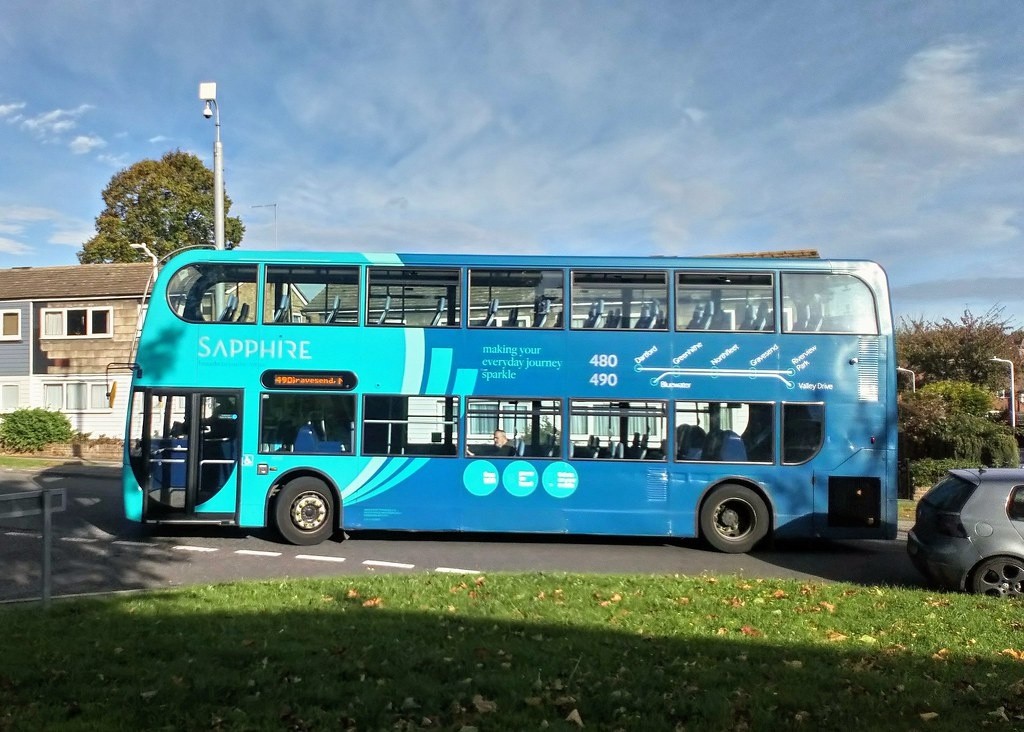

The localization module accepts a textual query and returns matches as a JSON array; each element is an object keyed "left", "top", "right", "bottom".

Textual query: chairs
[
  {"left": 216, "top": 294, "right": 825, "bottom": 331},
  {"left": 512, "top": 432, "right": 668, "bottom": 460}
]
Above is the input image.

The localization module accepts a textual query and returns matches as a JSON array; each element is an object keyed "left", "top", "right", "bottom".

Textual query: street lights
[
  {"left": 896, "top": 366, "right": 916, "bottom": 394},
  {"left": 129, "top": 242, "right": 158, "bottom": 283},
  {"left": 989, "top": 355, "right": 1016, "bottom": 437},
  {"left": 199, "top": 81, "right": 224, "bottom": 417}
]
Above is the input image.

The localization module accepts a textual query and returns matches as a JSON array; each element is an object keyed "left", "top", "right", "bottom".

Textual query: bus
[{"left": 104, "top": 242, "right": 901, "bottom": 554}]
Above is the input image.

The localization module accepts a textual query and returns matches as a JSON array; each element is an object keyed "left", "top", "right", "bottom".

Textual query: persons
[
  {"left": 467, "top": 430, "right": 520, "bottom": 457},
  {"left": 200, "top": 395, "right": 240, "bottom": 439}
]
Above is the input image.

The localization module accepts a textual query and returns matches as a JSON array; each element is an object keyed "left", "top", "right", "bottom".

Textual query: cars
[{"left": 905, "top": 466, "right": 1024, "bottom": 600}]
